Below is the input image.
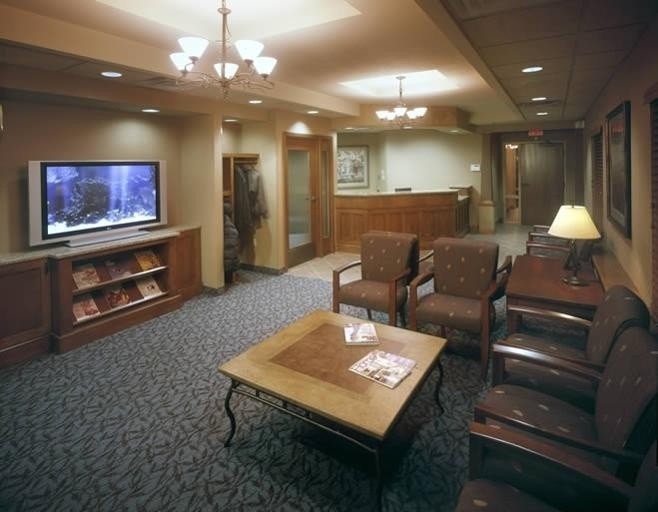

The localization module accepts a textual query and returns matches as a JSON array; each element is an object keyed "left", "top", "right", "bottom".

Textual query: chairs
[
  {"left": 505, "top": 253, "right": 606, "bottom": 338},
  {"left": 456, "top": 417, "right": 658, "bottom": 511},
  {"left": 525, "top": 231, "right": 574, "bottom": 268},
  {"left": 332, "top": 224, "right": 512, "bottom": 381},
  {"left": 528, "top": 222, "right": 573, "bottom": 244},
  {"left": 494, "top": 285, "right": 649, "bottom": 368},
  {"left": 471, "top": 319, "right": 657, "bottom": 464}
]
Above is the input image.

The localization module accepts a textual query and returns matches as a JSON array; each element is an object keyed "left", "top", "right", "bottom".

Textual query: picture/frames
[
  {"left": 336, "top": 144, "right": 370, "bottom": 189},
  {"left": 600, "top": 100, "right": 633, "bottom": 242}
]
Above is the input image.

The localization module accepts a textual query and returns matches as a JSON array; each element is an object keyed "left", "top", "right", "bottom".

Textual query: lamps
[
  {"left": 374, "top": 74, "right": 428, "bottom": 129},
  {"left": 548, "top": 201, "right": 604, "bottom": 288},
  {"left": 167, "top": 0, "right": 278, "bottom": 105}
]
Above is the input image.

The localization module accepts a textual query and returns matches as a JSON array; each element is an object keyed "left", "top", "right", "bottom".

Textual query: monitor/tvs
[{"left": 28, "top": 159, "right": 169, "bottom": 248}]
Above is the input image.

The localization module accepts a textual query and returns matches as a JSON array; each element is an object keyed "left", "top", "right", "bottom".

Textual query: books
[
  {"left": 348, "top": 349, "right": 417, "bottom": 389},
  {"left": 135, "top": 276, "right": 163, "bottom": 300},
  {"left": 102, "top": 285, "right": 132, "bottom": 310},
  {"left": 104, "top": 257, "right": 132, "bottom": 281},
  {"left": 134, "top": 250, "right": 162, "bottom": 272},
  {"left": 343, "top": 323, "right": 380, "bottom": 346},
  {"left": 71, "top": 294, "right": 101, "bottom": 322},
  {"left": 72, "top": 263, "right": 101, "bottom": 290}
]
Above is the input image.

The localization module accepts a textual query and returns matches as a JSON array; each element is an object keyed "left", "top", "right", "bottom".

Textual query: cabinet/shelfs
[
  {"left": 220, "top": 153, "right": 261, "bottom": 284},
  {"left": 46, "top": 228, "right": 183, "bottom": 357},
  {"left": 162, "top": 223, "right": 203, "bottom": 311},
  {"left": 0, "top": 251, "right": 54, "bottom": 369}
]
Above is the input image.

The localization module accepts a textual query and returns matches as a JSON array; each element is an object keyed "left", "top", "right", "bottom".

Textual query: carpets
[{"left": 1, "top": 271, "right": 508, "bottom": 511}]
